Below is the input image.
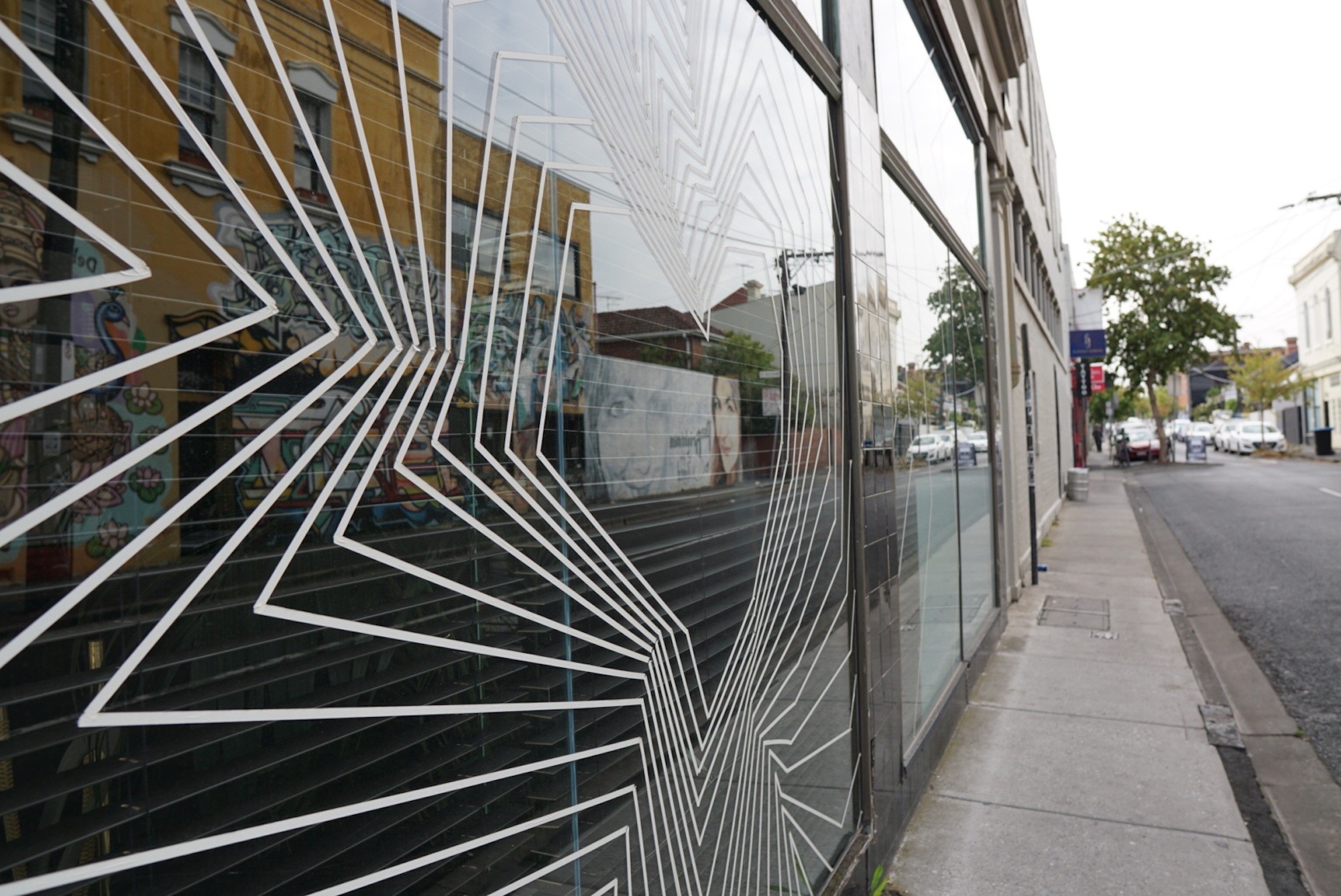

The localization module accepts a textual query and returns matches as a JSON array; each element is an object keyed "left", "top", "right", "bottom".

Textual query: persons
[
  {"left": 710, "top": 377, "right": 743, "bottom": 486},
  {"left": 1092, "top": 425, "right": 1103, "bottom": 451},
  {"left": 585, "top": 355, "right": 677, "bottom": 506}
]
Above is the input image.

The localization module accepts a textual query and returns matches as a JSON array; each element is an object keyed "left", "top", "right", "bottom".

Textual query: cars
[
  {"left": 1104, "top": 417, "right": 1288, "bottom": 463},
  {"left": 906, "top": 427, "right": 988, "bottom": 465}
]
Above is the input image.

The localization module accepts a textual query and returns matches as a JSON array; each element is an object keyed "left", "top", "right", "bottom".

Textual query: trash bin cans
[
  {"left": 1068, "top": 467, "right": 1089, "bottom": 501},
  {"left": 1314, "top": 429, "right": 1332, "bottom": 457}
]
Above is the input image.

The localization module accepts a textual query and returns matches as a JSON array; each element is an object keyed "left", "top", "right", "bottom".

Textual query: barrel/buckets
[{"left": 1067, "top": 467, "right": 1089, "bottom": 501}]
